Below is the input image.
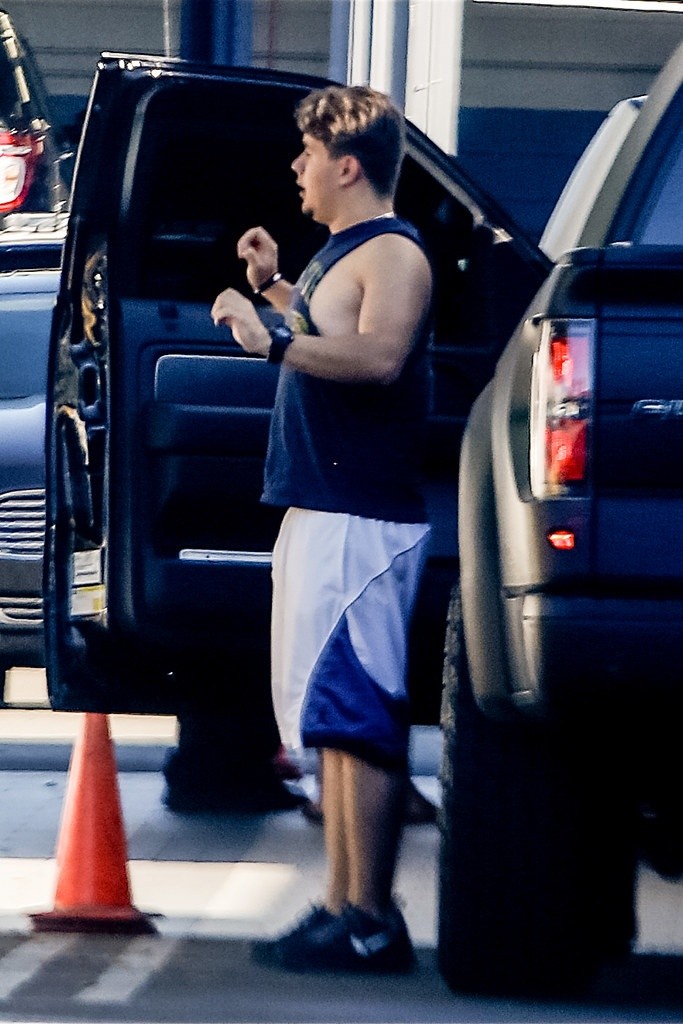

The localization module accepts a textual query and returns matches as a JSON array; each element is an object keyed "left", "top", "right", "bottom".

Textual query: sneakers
[{"left": 249, "top": 907, "right": 418, "bottom": 976}]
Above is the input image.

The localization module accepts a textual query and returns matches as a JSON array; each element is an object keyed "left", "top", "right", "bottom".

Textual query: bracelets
[{"left": 253, "top": 272, "right": 283, "bottom": 294}]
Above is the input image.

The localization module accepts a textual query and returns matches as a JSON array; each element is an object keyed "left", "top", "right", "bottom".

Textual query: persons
[{"left": 211, "top": 86, "right": 432, "bottom": 976}]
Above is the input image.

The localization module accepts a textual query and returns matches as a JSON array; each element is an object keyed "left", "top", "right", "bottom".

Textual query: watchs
[{"left": 266, "top": 324, "right": 294, "bottom": 364}]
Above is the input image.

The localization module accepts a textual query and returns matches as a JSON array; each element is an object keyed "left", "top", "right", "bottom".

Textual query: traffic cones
[{"left": 27, "top": 710, "right": 167, "bottom": 936}]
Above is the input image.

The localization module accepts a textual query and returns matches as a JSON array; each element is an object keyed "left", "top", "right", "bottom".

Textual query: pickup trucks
[{"left": 41, "top": 33, "right": 682, "bottom": 1010}]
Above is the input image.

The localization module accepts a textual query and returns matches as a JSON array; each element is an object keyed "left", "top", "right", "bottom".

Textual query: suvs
[{"left": 1, "top": 7, "right": 78, "bottom": 711}]
